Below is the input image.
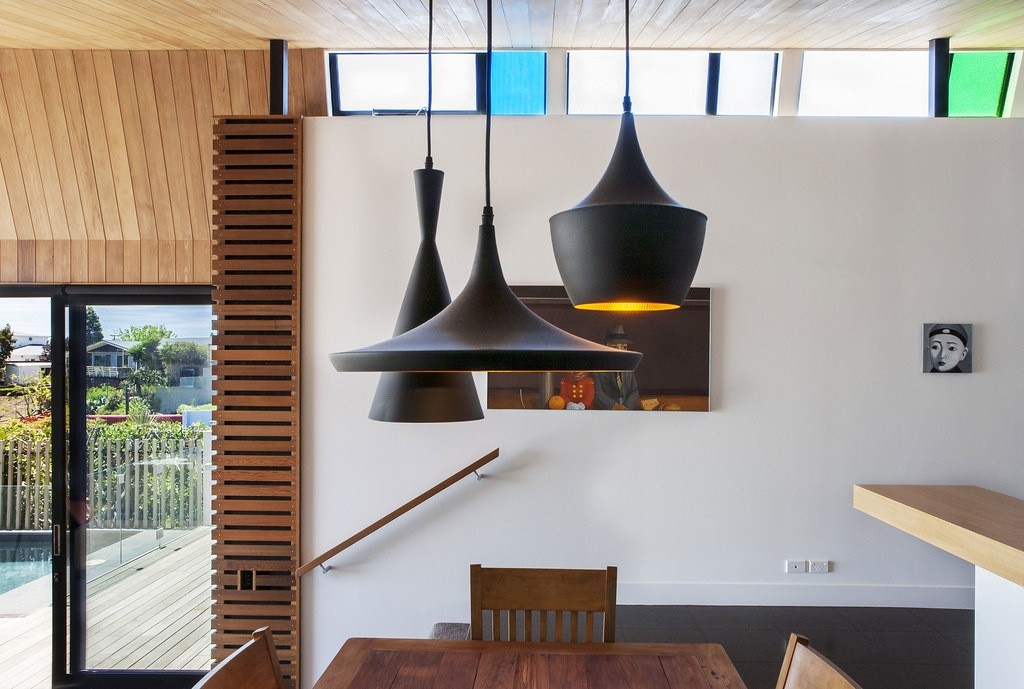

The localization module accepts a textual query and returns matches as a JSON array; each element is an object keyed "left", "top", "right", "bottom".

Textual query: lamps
[
  {"left": 367, "top": 0, "right": 485, "bottom": 424},
  {"left": 549, "top": 1, "right": 708, "bottom": 312},
  {"left": 329, "top": 0, "right": 643, "bottom": 371}
]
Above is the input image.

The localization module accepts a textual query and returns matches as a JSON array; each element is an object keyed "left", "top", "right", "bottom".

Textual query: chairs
[
  {"left": 191, "top": 626, "right": 285, "bottom": 689},
  {"left": 470, "top": 564, "right": 617, "bottom": 643},
  {"left": 775, "top": 633, "right": 862, "bottom": 689}
]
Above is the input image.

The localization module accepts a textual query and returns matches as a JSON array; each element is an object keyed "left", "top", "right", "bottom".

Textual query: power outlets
[{"left": 808, "top": 560, "right": 829, "bottom": 573}]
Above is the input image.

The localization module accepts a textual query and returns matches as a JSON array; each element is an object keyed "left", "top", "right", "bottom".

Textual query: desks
[
  {"left": 853, "top": 485, "right": 1024, "bottom": 586},
  {"left": 311, "top": 638, "right": 749, "bottom": 689}
]
